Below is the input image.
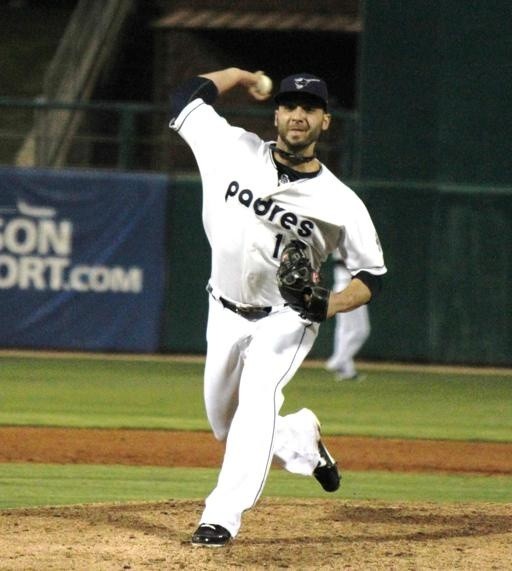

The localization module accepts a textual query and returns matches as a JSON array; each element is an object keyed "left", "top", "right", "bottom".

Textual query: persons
[
  {"left": 324, "top": 245, "right": 371, "bottom": 381},
  {"left": 169, "top": 68, "right": 386, "bottom": 547}
]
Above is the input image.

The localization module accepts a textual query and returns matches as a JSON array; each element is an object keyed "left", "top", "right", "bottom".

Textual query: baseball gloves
[{"left": 277, "top": 238, "right": 331, "bottom": 322}]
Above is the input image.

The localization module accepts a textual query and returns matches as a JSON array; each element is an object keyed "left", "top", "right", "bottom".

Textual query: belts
[{"left": 207, "top": 285, "right": 271, "bottom": 319}]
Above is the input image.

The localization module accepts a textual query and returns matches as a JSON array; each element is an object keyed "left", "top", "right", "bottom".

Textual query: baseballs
[{"left": 258, "top": 75, "right": 272, "bottom": 95}]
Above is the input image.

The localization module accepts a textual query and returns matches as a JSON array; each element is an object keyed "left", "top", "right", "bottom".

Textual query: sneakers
[
  {"left": 305, "top": 407, "right": 340, "bottom": 491},
  {"left": 192, "top": 522, "right": 230, "bottom": 548}
]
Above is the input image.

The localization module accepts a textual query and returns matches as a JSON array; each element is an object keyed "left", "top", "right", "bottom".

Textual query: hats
[{"left": 274, "top": 74, "right": 328, "bottom": 108}]
